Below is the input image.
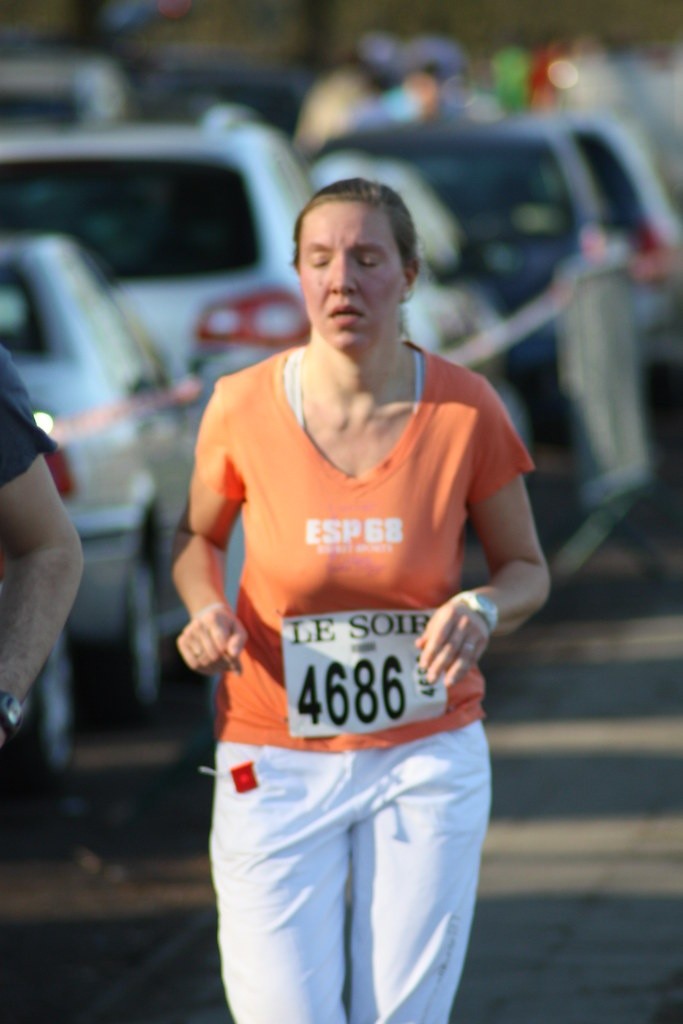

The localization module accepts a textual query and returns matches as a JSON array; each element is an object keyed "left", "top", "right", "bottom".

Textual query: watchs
[
  {"left": 452, "top": 591, "right": 498, "bottom": 633},
  {"left": 0, "top": 690, "right": 24, "bottom": 743}
]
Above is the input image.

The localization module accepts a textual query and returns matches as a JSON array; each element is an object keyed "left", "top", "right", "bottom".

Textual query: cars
[
  {"left": 1, "top": 229, "right": 199, "bottom": 724},
  {"left": 1, "top": 44, "right": 683, "bottom": 464}
]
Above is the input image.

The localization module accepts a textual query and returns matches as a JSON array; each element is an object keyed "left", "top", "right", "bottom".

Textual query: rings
[
  {"left": 193, "top": 649, "right": 203, "bottom": 657},
  {"left": 462, "top": 642, "right": 475, "bottom": 652}
]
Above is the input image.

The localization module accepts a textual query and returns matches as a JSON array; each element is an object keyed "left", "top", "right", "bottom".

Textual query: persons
[
  {"left": 0, "top": 346, "right": 83, "bottom": 753},
  {"left": 293, "top": 37, "right": 565, "bottom": 160},
  {"left": 172, "top": 178, "right": 549, "bottom": 1024}
]
